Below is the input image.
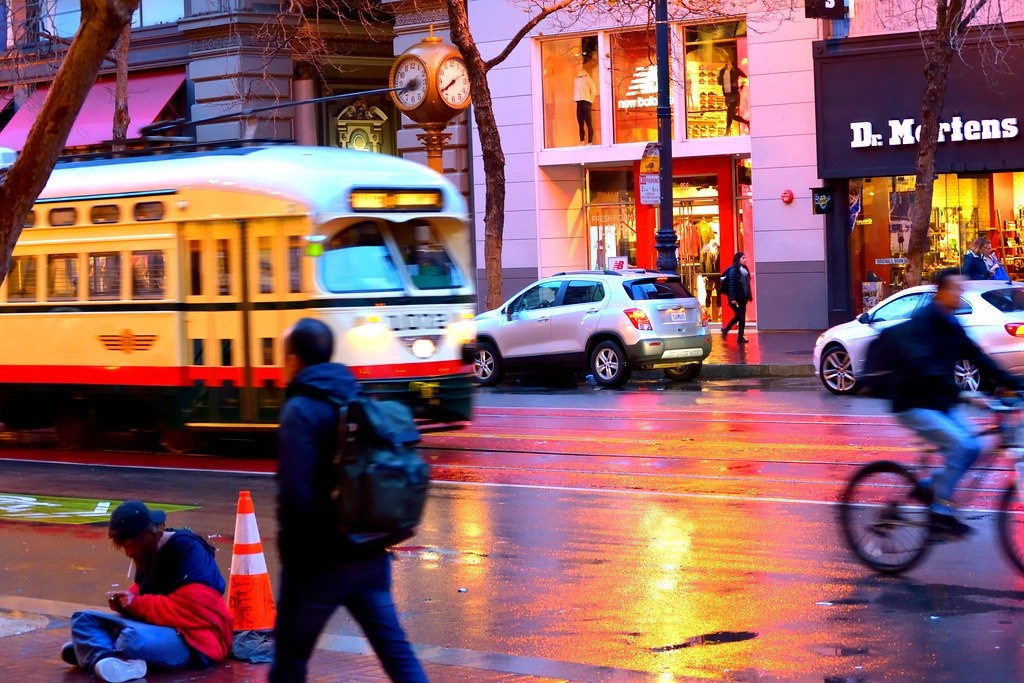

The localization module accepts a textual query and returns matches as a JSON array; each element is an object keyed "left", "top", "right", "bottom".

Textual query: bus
[{"left": 0, "top": 136, "right": 484, "bottom": 439}]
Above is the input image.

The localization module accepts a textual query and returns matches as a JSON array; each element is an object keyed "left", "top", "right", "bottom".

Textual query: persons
[
  {"left": 700, "top": 240, "right": 723, "bottom": 322},
  {"left": 575, "top": 70, "right": 596, "bottom": 145},
  {"left": 885, "top": 266, "right": 1024, "bottom": 541},
  {"left": 718, "top": 252, "right": 752, "bottom": 343},
  {"left": 263, "top": 318, "right": 432, "bottom": 683},
  {"left": 62, "top": 501, "right": 235, "bottom": 683},
  {"left": 717, "top": 61, "right": 751, "bottom": 138},
  {"left": 962, "top": 237, "right": 1005, "bottom": 281}
]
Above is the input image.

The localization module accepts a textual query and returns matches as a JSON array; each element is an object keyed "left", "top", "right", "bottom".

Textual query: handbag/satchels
[{"left": 993, "top": 266, "right": 1010, "bottom": 281}]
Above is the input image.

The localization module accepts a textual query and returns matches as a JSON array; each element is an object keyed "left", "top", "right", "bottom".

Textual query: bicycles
[{"left": 833, "top": 389, "right": 1024, "bottom": 574}]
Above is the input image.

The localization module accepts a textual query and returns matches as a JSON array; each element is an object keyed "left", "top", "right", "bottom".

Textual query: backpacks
[
  {"left": 719, "top": 268, "right": 734, "bottom": 295},
  {"left": 856, "top": 319, "right": 923, "bottom": 398},
  {"left": 288, "top": 387, "right": 428, "bottom": 536}
]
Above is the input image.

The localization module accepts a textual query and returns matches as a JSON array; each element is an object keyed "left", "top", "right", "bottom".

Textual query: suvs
[{"left": 461, "top": 268, "right": 713, "bottom": 388}]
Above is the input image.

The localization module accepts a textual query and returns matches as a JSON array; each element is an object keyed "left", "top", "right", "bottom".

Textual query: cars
[{"left": 811, "top": 280, "right": 1022, "bottom": 395}]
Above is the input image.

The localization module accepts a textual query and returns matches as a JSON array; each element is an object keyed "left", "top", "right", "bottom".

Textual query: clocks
[
  {"left": 436, "top": 52, "right": 474, "bottom": 110},
  {"left": 387, "top": 52, "right": 431, "bottom": 112}
]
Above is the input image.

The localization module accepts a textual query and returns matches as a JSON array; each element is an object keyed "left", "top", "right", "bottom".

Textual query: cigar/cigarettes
[{"left": 127, "top": 557, "right": 134, "bottom": 577}]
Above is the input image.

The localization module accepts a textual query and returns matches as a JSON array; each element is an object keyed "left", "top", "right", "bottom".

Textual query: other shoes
[
  {"left": 721, "top": 328, "right": 727, "bottom": 339},
  {"left": 926, "top": 513, "right": 973, "bottom": 534},
  {"left": 60, "top": 643, "right": 79, "bottom": 665},
  {"left": 910, "top": 484, "right": 937, "bottom": 506},
  {"left": 737, "top": 337, "right": 748, "bottom": 343},
  {"left": 94, "top": 658, "right": 147, "bottom": 683}
]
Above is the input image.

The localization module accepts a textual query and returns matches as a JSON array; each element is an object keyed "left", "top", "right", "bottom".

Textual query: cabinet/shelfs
[
  {"left": 996, "top": 205, "right": 1024, "bottom": 281},
  {"left": 927, "top": 232, "right": 948, "bottom": 254}
]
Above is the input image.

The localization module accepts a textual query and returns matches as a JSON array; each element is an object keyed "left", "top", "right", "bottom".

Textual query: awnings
[{"left": 1, "top": 65, "right": 190, "bottom": 153}]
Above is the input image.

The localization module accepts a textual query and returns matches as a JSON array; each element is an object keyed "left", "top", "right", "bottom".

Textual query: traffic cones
[{"left": 222, "top": 488, "right": 275, "bottom": 634}]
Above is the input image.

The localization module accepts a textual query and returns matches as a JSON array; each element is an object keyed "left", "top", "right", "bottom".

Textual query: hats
[{"left": 109, "top": 501, "right": 165, "bottom": 534}]
